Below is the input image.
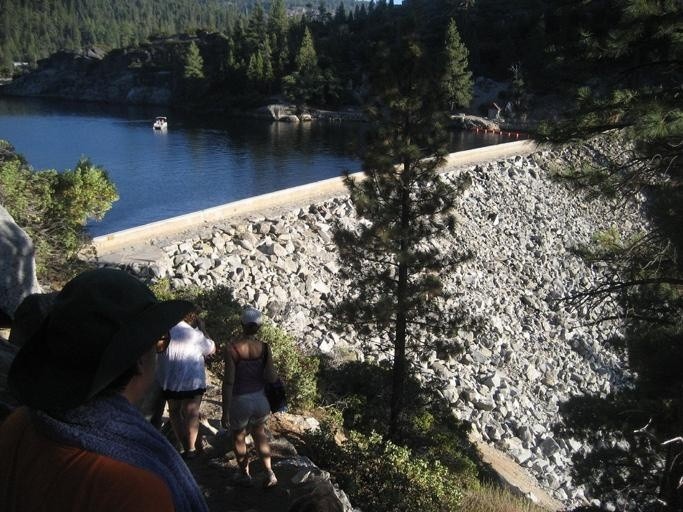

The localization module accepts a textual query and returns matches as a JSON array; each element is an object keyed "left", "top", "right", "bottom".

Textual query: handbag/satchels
[{"left": 264, "top": 378, "right": 287, "bottom": 413}]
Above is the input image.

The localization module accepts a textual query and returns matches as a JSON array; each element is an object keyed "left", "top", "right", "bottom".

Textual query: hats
[
  {"left": 241, "top": 309, "right": 262, "bottom": 327},
  {"left": 8, "top": 267, "right": 195, "bottom": 412}
]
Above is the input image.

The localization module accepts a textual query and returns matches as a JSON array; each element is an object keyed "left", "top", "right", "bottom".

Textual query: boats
[
  {"left": 151, "top": 115, "right": 169, "bottom": 136},
  {"left": 298, "top": 111, "right": 312, "bottom": 122}
]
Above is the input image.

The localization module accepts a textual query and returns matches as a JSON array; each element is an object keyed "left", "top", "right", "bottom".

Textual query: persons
[
  {"left": 219, "top": 305, "right": 282, "bottom": 488},
  {"left": 0, "top": 268, "right": 210, "bottom": 511},
  {"left": 157, "top": 319, "right": 217, "bottom": 459}
]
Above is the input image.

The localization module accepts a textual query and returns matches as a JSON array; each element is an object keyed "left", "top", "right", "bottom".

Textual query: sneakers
[
  {"left": 177, "top": 448, "right": 200, "bottom": 458},
  {"left": 233, "top": 475, "right": 251, "bottom": 482},
  {"left": 262, "top": 471, "right": 278, "bottom": 489}
]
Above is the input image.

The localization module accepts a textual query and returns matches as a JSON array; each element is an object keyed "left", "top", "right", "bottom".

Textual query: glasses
[{"left": 156, "top": 331, "right": 172, "bottom": 354}]
